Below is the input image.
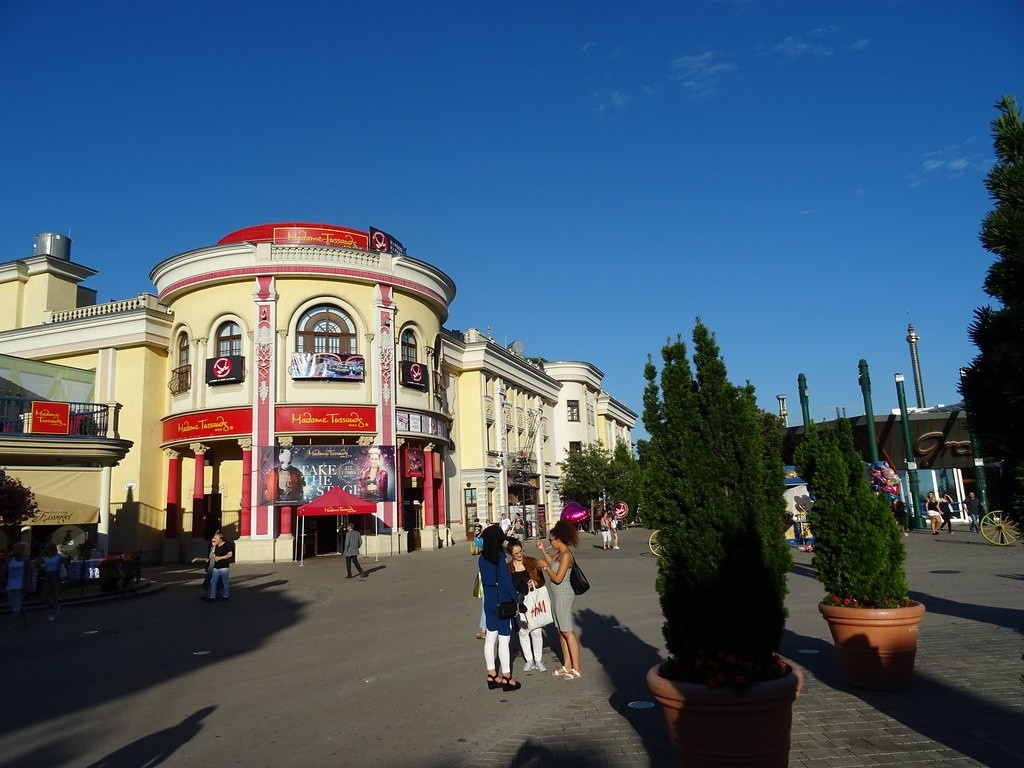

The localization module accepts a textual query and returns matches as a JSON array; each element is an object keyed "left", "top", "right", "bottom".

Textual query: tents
[{"left": 295, "top": 487, "right": 377, "bottom": 565}]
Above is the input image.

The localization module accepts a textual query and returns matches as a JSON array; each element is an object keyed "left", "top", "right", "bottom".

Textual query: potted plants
[
  {"left": 794, "top": 418, "right": 926, "bottom": 693},
  {"left": 79, "top": 417, "right": 99, "bottom": 436},
  {"left": 643, "top": 318, "right": 804, "bottom": 768}
]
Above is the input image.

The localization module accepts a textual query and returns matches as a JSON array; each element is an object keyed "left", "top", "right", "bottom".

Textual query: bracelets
[{"left": 544, "top": 566, "right": 549, "bottom": 570}]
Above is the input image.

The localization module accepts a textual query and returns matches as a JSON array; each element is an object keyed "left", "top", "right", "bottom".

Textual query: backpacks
[{"left": 563, "top": 550, "right": 590, "bottom": 595}]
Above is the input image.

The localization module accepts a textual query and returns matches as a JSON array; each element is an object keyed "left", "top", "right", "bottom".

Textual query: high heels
[
  {"left": 487, "top": 674, "right": 502, "bottom": 689},
  {"left": 502, "top": 676, "right": 521, "bottom": 691}
]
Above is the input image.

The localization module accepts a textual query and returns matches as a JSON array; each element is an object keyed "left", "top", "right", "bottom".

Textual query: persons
[
  {"left": 893, "top": 498, "right": 908, "bottom": 536},
  {"left": 359, "top": 452, "right": 388, "bottom": 501},
  {"left": 962, "top": 492, "right": 985, "bottom": 532},
  {"left": 601, "top": 510, "right": 620, "bottom": 549},
  {"left": 473, "top": 513, "right": 524, "bottom": 555},
  {"left": 343, "top": 524, "right": 366, "bottom": 578},
  {"left": 200, "top": 535, "right": 232, "bottom": 602},
  {"left": 265, "top": 450, "right": 304, "bottom": 502},
  {"left": 926, "top": 492, "right": 953, "bottom": 534},
  {"left": 2, "top": 543, "right": 32, "bottom": 630},
  {"left": 37, "top": 543, "right": 65, "bottom": 622},
  {"left": 537, "top": 523, "right": 582, "bottom": 679},
  {"left": 413, "top": 451, "right": 422, "bottom": 471},
  {"left": 477, "top": 525, "right": 546, "bottom": 691}
]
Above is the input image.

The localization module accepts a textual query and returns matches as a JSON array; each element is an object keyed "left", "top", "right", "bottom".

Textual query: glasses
[
  {"left": 549, "top": 537, "right": 559, "bottom": 543},
  {"left": 512, "top": 548, "right": 524, "bottom": 556}
]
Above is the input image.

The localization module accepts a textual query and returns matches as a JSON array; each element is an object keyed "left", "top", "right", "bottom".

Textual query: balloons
[
  {"left": 869, "top": 461, "right": 899, "bottom": 501},
  {"left": 560, "top": 502, "right": 590, "bottom": 523}
]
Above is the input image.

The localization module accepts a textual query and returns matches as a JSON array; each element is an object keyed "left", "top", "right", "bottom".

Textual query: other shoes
[
  {"left": 613, "top": 546, "right": 620, "bottom": 549},
  {"left": 345, "top": 576, "right": 351, "bottom": 578},
  {"left": 524, "top": 660, "right": 535, "bottom": 671},
  {"left": 206, "top": 598, "right": 215, "bottom": 602},
  {"left": 476, "top": 633, "right": 486, "bottom": 639},
  {"left": 4, "top": 624, "right": 15, "bottom": 630},
  {"left": 476, "top": 553, "right": 480, "bottom": 555},
  {"left": 933, "top": 526, "right": 979, "bottom": 535},
  {"left": 216, "top": 592, "right": 222, "bottom": 599},
  {"left": 47, "top": 615, "right": 56, "bottom": 622},
  {"left": 200, "top": 595, "right": 207, "bottom": 599},
  {"left": 55, "top": 606, "right": 60, "bottom": 616},
  {"left": 535, "top": 662, "right": 547, "bottom": 672},
  {"left": 220, "top": 597, "right": 229, "bottom": 603},
  {"left": 360, "top": 572, "right": 365, "bottom": 578},
  {"left": 24, "top": 613, "right": 32, "bottom": 627}
]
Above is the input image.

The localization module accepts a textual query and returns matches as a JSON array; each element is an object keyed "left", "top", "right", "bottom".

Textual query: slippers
[
  {"left": 552, "top": 666, "right": 569, "bottom": 675},
  {"left": 563, "top": 669, "right": 582, "bottom": 680}
]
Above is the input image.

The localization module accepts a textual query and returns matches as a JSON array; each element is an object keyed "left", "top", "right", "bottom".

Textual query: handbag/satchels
[
  {"left": 473, "top": 573, "right": 483, "bottom": 599},
  {"left": 497, "top": 601, "right": 517, "bottom": 620},
  {"left": 600, "top": 520, "right": 608, "bottom": 531},
  {"left": 519, "top": 580, "right": 554, "bottom": 635},
  {"left": 948, "top": 503, "right": 953, "bottom": 512}
]
